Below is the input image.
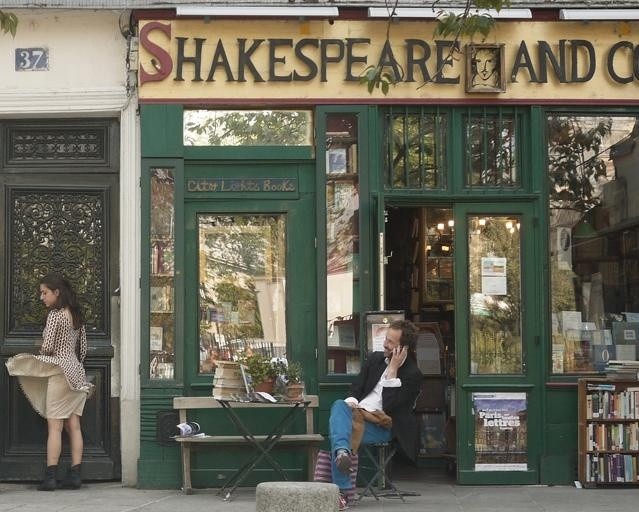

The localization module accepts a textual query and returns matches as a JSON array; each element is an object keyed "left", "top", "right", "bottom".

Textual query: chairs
[{"left": 356, "top": 386, "right": 424, "bottom": 506}]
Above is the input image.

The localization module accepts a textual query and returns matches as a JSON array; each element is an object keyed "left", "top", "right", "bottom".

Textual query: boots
[
  {"left": 37, "top": 465, "right": 58, "bottom": 490},
  {"left": 58, "top": 464, "right": 82, "bottom": 488}
]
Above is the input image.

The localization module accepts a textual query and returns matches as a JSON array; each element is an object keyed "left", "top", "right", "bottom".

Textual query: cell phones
[{"left": 389, "top": 347, "right": 403, "bottom": 362}]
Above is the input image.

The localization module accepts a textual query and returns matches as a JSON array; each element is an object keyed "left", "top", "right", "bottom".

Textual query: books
[
  {"left": 451, "top": 384, "right": 456, "bottom": 416},
  {"left": 200, "top": 299, "right": 285, "bottom": 401},
  {"left": 327, "top": 208, "right": 456, "bottom": 376},
  {"left": 149, "top": 241, "right": 178, "bottom": 380},
  {"left": 446, "top": 384, "right": 452, "bottom": 417},
  {"left": 551, "top": 119, "right": 639, "bottom": 484},
  {"left": 417, "top": 411, "right": 448, "bottom": 447},
  {"left": 419, "top": 447, "right": 448, "bottom": 454}
]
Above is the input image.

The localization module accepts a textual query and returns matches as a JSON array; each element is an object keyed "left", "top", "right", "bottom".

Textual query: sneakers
[
  {"left": 334, "top": 449, "right": 354, "bottom": 474},
  {"left": 339, "top": 496, "right": 349, "bottom": 510}
]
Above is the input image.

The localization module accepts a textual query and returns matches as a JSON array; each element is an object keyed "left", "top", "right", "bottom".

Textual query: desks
[{"left": 214, "top": 399, "right": 312, "bottom": 506}]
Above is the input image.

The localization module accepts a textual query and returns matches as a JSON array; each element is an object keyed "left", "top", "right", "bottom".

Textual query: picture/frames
[{"left": 463, "top": 43, "right": 507, "bottom": 96}]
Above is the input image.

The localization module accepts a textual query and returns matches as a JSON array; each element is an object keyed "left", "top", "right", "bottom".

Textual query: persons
[
  {"left": 326, "top": 320, "right": 423, "bottom": 512},
  {"left": 5, "top": 269, "right": 99, "bottom": 491}
]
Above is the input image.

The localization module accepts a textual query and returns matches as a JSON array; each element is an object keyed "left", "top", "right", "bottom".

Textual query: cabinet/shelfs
[
  {"left": 409, "top": 206, "right": 455, "bottom": 472},
  {"left": 575, "top": 375, "right": 639, "bottom": 491}
]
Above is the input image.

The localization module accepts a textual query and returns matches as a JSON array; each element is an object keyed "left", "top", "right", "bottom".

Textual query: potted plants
[{"left": 198, "top": 279, "right": 306, "bottom": 399}]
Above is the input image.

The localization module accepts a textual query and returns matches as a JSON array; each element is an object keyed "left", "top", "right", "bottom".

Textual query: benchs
[{"left": 169, "top": 395, "right": 320, "bottom": 496}]
